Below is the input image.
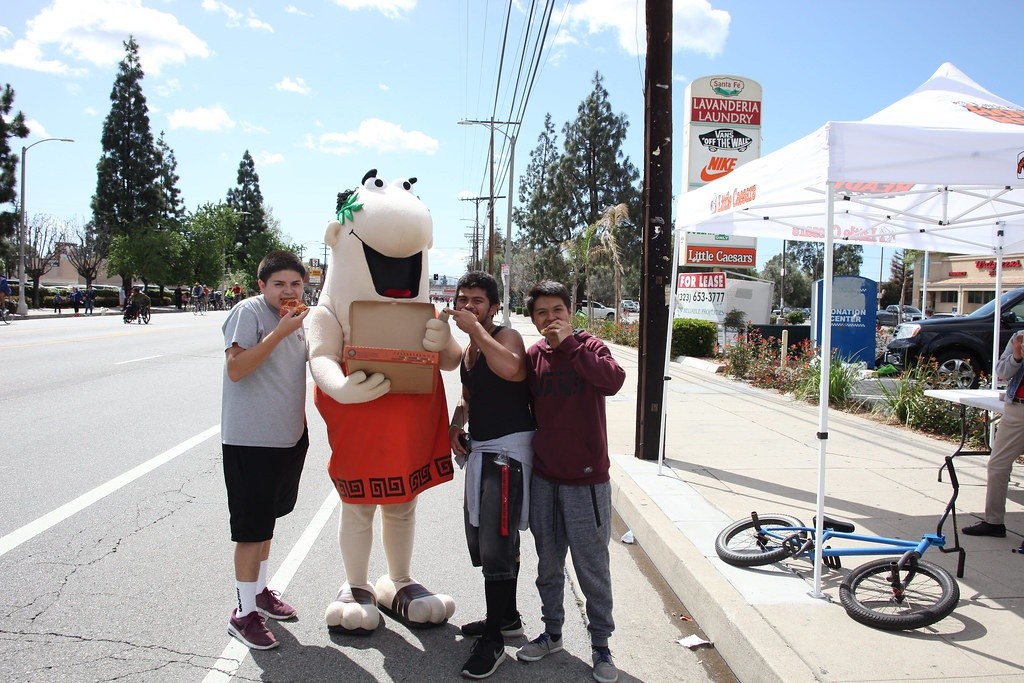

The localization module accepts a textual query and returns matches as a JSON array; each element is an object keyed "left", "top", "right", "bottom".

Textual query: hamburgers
[{"left": 540, "top": 327, "right": 551, "bottom": 334}]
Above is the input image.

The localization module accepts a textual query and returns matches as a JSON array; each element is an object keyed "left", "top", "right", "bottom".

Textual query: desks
[{"left": 923, "top": 388, "right": 1010, "bottom": 483}]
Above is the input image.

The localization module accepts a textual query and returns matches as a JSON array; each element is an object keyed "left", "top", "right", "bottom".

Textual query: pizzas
[
  {"left": 280, "top": 299, "right": 307, "bottom": 316},
  {"left": 443, "top": 309, "right": 480, "bottom": 318}
]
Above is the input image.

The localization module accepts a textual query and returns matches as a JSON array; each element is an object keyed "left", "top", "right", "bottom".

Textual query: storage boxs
[{"left": 343, "top": 299, "right": 440, "bottom": 397}]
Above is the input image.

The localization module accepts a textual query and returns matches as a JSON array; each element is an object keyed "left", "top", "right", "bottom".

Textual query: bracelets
[{"left": 449, "top": 425, "right": 459, "bottom": 432}]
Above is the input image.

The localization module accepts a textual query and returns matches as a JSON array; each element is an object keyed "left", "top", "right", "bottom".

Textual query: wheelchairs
[{"left": 122, "top": 300, "right": 151, "bottom": 325}]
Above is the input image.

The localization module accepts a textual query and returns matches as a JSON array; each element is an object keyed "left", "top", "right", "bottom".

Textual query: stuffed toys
[{"left": 306, "top": 169, "right": 458, "bottom": 634}]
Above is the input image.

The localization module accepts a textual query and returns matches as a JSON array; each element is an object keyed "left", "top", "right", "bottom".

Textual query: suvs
[{"left": 884, "top": 288, "right": 1024, "bottom": 388}]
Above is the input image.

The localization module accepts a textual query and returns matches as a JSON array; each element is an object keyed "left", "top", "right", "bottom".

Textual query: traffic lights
[{"left": 433, "top": 274, "right": 439, "bottom": 280}]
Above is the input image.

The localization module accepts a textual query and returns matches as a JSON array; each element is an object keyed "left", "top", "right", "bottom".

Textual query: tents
[{"left": 655, "top": 62, "right": 1024, "bottom": 598}]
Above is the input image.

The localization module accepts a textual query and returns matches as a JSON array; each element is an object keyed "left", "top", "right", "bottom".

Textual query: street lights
[
  {"left": 221, "top": 211, "right": 251, "bottom": 304},
  {"left": 15, "top": 138, "right": 75, "bottom": 317},
  {"left": 457, "top": 121, "right": 517, "bottom": 327},
  {"left": 460, "top": 218, "right": 485, "bottom": 271}
]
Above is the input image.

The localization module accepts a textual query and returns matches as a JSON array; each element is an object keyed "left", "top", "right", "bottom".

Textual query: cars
[
  {"left": 774, "top": 304, "right": 968, "bottom": 327},
  {"left": 583, "top": 299, "right": 639, "bottom": 318}
]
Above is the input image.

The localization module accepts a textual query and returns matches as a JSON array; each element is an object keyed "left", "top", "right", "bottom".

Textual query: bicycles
[
  {"left": 189, "top": 293, "right": 237, "bottom": 315},
  {"left": 716, "top": 456, "right": 967, "bottom": 629},
  {"left": 0, "top": 302, "right": 15, "bottom": 325}
]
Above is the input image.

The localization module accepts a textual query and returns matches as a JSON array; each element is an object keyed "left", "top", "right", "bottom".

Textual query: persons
[
  {"left": 962, "top": 330, "right": 1024, "bottom": 556},
  {"left": 175, "top": 285, "right": 183, "bottom": 309},
  {"left": 182, "top": 293, "right": 188, "bottom": 308},
  {"left": 70, "top": 287, "right": 83, "bottom": 313},
  {"left": 54, "top": 293, "right": 62, "bottom": 313},
  {"left": 516, "top": 281, "right": 626, "bottom": 683},
  {"left": 83, "top": 285, "right": 95, "bottom": 316},
  {"left": 0, "top": 274, "right": 11, "bottom": 314},
  {"left": 429, "top": 294, "right": 453, "bottom": 302},
  {"left": 305, "top": 289, "right": 321, "bottom": 303},
  {"left": 497, "top": 301, "right": 503, "bottom": 314},
  {"left": 445, "top": 272, "right": 536, "bottom": 680},
  {"left": 220, "top": 249, "right": 308, "bottom": 650},
  {"left": 189, "top": 282, "right": 220, "bottom": 310},
  {"left": 120, "top": 287, "right": 150, "bottom": 320},
  {"left": 224, "top": 282, "right": 242, "bottom": 306}
]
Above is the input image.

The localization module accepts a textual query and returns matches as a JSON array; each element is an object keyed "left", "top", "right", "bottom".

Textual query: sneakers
[
  {"left": 516, "top": 632, "right": 564, "bottom": 661},
  {"left": 227, "top": 608, "right": 280, "bottom": 650},
  {"left": 461, "top": 612, "right": 524, "bottom": 638},
  {"left": 461, "top": 632, "right": 506, "bottom": 678},
  {"left": 256, "top": 587, "right": 296, "bottom": 619},
  {"left": 961, "top": 520, "right": 1006, "bottom": 537},
  {"left": 591, "top": 647, "right": 619, "bottom": 683}
]
